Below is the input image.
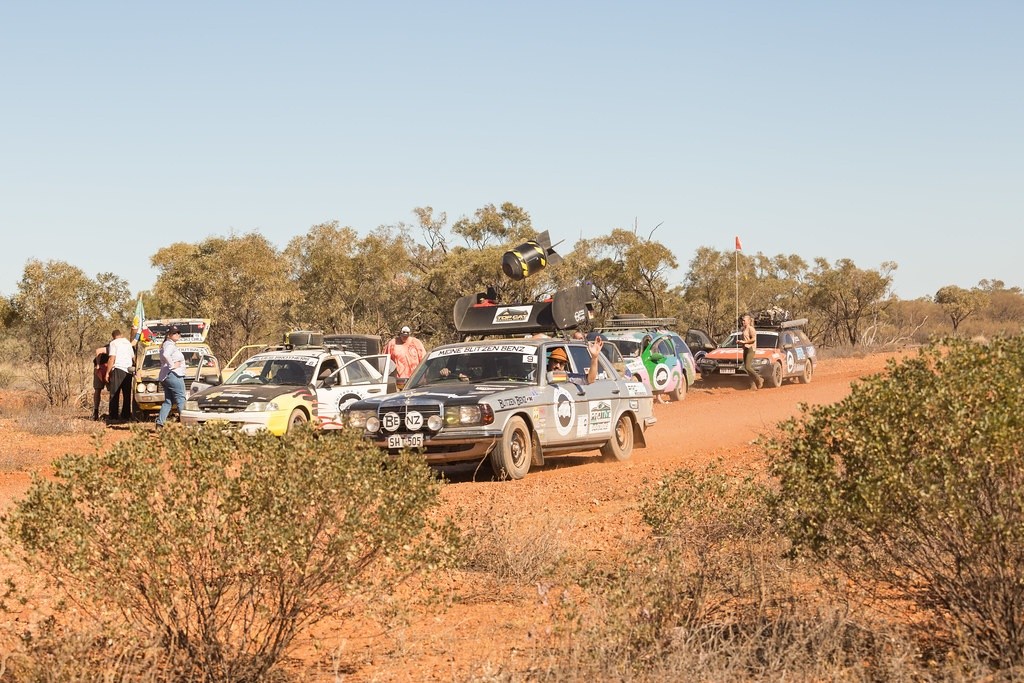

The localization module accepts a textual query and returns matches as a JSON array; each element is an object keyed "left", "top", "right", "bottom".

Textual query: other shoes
[
  {"left": 757, "top": 378, "right": 764, "bottom": 388},
  {"left": 749, "top": 385, "right": 758, "bottom": 391},
  {"left": 93, "top": 415, "right": 99, "bottom": 421}
]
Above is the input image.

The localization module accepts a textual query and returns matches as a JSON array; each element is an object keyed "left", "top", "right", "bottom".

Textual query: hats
[
  {"left": 399, "top": 326, "right": 412, "bottom": 336},
  {"left": 547, "top": 349, "right": 569, "bottom": 362},
  {"left": 170, "top": 325, "right": 181, "bottom": 332}
]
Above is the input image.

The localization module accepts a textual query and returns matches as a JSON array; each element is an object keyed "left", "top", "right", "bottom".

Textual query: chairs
[{"left": 275, "top": 368, "right": 289, "bottom": 383}]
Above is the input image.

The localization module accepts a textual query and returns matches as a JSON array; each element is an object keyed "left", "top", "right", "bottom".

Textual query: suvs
[
  {"left": 591, "top": 312, "right": 697, "bottom": 403},
  {"left": 682, "top": 318, "right": 817, "bottom": 388},
  {"left": 342, "top": 282, "right": 657, "bottom": 483},
  {"left": 178, "top": 331, "right": 399, "bottom": 442},
  {"left": 125, "top": 316, "right": 273, "bottom": 424}
]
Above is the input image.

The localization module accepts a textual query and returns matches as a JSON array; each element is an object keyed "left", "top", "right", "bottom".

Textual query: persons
[
  {"left": 156, "top": 326, "right": 187, "bottom": 431},
  {"left": 384, "top": 326, "right": 427, "bottom": 390},
  {"left": 92, "top": 344, "right": 110, "bottom": 421},
  {"left": 106, "top": 330, "right": 135, "bottom": 420},
  {"left": 524, "top": 336, "right": 603, "bottom": 384},
  {"left": 736, "top": 314, "right": 764, "bottom": 391},
  {"left": 573, "top": 331, "right": 609, "bottom": 360},
  {"left": 440, "top": 362, "right": 483, "bottom": 381}
]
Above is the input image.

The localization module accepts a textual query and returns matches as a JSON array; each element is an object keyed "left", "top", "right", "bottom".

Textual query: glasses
[
  {"left": 553, "top": 360, "right": 566, "bottom": 366},
  {"left": 173, "top": 332, "right": 180, "bottom": 334}
]
